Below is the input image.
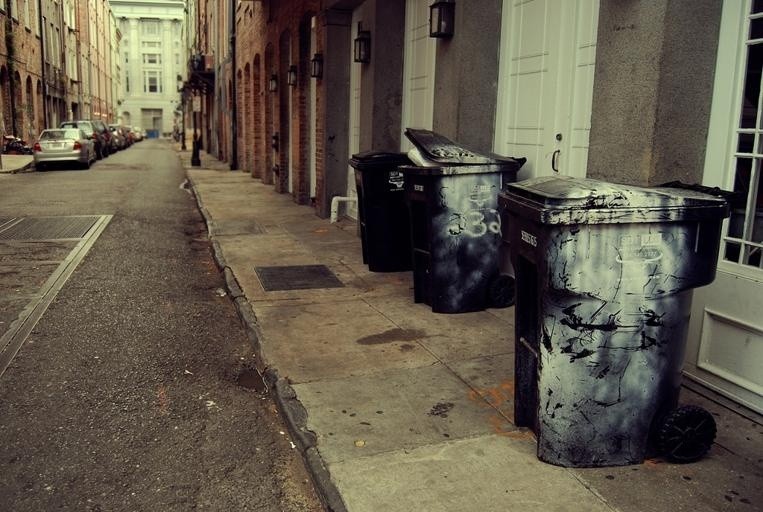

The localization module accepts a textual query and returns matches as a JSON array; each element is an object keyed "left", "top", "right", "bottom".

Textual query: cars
[
  {"left": 107, "top": 126, "right": 126, "bottom": 151},
  {"left": 87, "top": 121, "right": 116, "bottom": 157},
  {"left": 108, "top": 124, "right": 146, "bottom": 148},
  {"left": 33, "top": 128, "right": 96, "bottom": 170}
]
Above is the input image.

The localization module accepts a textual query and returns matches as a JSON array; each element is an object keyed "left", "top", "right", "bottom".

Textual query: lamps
[
  {"left": 349, "top": 27, "right": 374, "bottom": 66},
  {"left": 267, "top": 73, "right": 280, "bottom": 93},
  {"left": 307, "top": 52, "right": 326, "bottom": 80},
  {"left": 428, "top": 0, "right": 457, "bottom": 42},
  {"left": 284, "top": 63, "right": 298, "bottom": 87}
]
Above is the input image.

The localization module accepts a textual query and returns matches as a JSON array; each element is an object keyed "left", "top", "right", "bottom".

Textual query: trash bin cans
[
  {"left": 349, "top": 149, "right": 414, "bottom": 272},
  {"left": 499, "top": 178, "right": 738, "bottom": 469},
  {"left": 398, "top": 128, "right": 528, "bottom": 313}
]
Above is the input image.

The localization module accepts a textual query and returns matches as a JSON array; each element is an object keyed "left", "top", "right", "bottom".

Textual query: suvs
[{"left": 58, "top": 121, "right": 105, "bottom": 159}]
